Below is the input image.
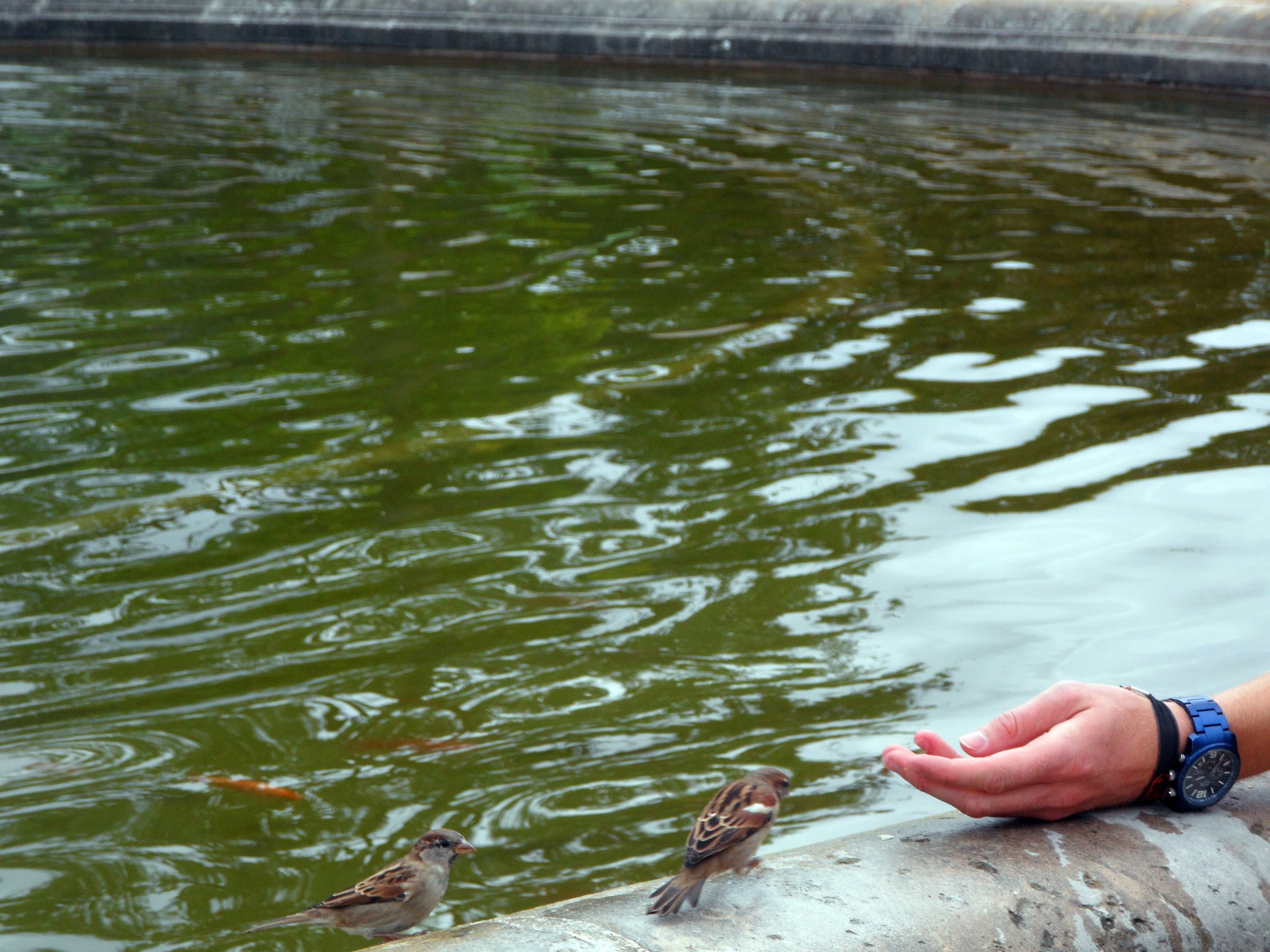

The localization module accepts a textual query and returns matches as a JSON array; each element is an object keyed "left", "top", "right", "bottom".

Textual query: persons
[{"left": 881, "top": 673, "right": 1270, "bottom": 820}]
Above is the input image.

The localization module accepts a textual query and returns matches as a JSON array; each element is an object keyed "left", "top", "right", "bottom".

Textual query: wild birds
[
  {"left": 244, "top": 828, "right": 478, "bottom": 943},
  {"left": 643, "top": 766, "right": 793, "bottom": 921}
]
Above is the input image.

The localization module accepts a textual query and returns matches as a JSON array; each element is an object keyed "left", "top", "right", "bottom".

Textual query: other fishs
[{"left": 182, "top": 771, "right": 303, "bottom": 800}]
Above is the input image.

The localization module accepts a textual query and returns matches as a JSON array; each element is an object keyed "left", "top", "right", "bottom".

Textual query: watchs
[{"left": 1161, "top": 694, "right": 1241, "bottom": 812}]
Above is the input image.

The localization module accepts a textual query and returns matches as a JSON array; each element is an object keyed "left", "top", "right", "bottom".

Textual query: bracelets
[{"left": 1121, "top": 685, "right": 1186, "bottom": 806}]
